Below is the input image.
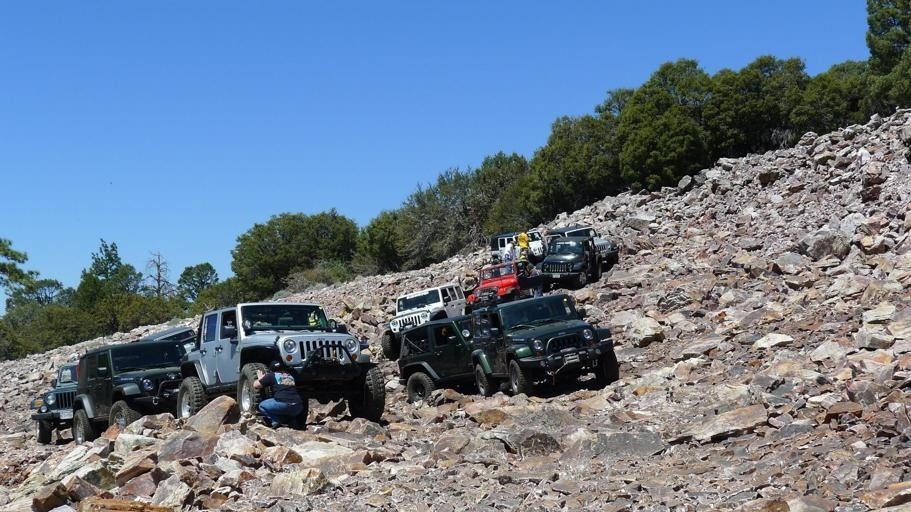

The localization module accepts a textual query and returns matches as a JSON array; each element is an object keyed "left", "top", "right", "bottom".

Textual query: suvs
[
  {"left": 540, "top": 235, "right": 605, "bottom": 289},
  {"left": 32, "top": 362, "right": 82, "bottom": 444},
  {"left": 489, "top": 229, "right": 546, "bottom": 264},
  {"left": 383, "top": 282, "right": 474, "bottom": 361},
  {"left": 547, "top": 223, "right": 619, "bottom": 270},
  {"left": 71, "top": 337, "right": 183, "bottom": 446},
  {"left": 470, "top": 260, "right": 540, "bottom": 308},
  {"left": 472, "top": 293, "right": 619, "bottom": 400},
  {"left": 399, "top": 315, "right": 501, "bottom": 401},
  {"left": 137, "top": 327, "right": 197, "bottom": 357},
  {"left": 180, "top": 301, "right": 389, "bottom": 426}
]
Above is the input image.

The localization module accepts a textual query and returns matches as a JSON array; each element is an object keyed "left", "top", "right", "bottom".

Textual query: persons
[
  {"left": 491, "top": 229, "right": 544, "bottom": 299},
  {"left": 253, "top": 360, "right": 305, "bottom": 430}
]
